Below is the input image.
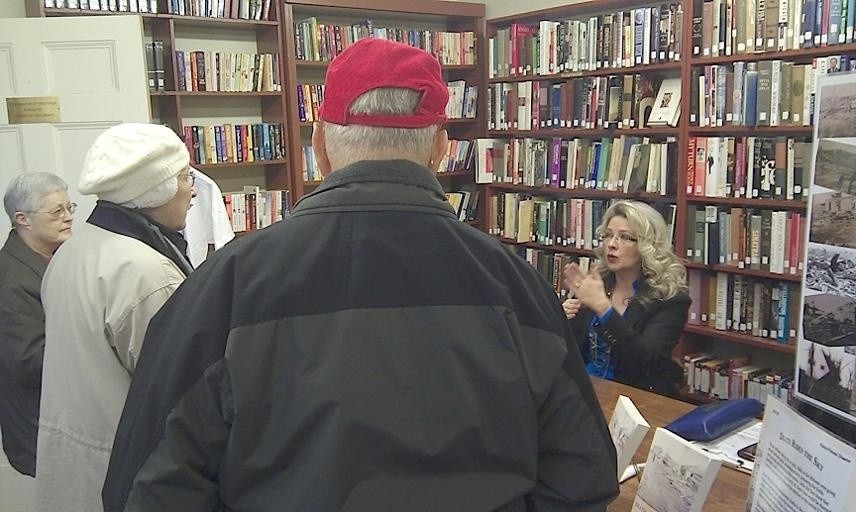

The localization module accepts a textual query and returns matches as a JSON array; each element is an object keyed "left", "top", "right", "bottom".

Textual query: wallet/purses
[{"left": 664, "top": 397, "right": 765, "bottom": 442}]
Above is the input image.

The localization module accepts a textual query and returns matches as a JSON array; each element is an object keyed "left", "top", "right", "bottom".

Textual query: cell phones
[{"left": 737, "top": 443, "right": 757, "bottom": 462}]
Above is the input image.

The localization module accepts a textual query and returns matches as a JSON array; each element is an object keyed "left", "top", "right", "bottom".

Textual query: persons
[
  {"left": 29, "top": 121, "right": 199, "bottom": 511},
  {"left": 96, "top": 29, "right": 627, "bottom": 509},
  {"left": 0, "top": 168, "right": 79, "bottom": 512}
]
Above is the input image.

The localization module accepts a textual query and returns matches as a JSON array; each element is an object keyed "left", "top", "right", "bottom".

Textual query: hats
[
  {"left": 320, "top": 37, "right": 453, "bottom": 132},
  {"left": 76, "top": 120, "right": 192, "bottom": 206}
]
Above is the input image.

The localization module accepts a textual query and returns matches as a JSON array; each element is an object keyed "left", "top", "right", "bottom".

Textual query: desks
[{"left": 588, "top": 371, "right": 751, "bottom": 512}]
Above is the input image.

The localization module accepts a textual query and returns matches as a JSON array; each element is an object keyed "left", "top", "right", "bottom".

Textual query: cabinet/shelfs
[
  {"left": 487, "top": 0, "right": 856, "bottom": 419},
  {"left": 23, "top": 0, "right": 487, "bottom": 258}
]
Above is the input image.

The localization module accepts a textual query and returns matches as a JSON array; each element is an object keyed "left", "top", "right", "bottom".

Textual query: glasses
[
  {"left": 173, "top": 169, "right": 197, "bottom": 188},
  {"left": 22, "top": 202, "right": 78, "bottom": 219},
  {"left": 600, "top": 229, "right": 640, "bottom": 243}
]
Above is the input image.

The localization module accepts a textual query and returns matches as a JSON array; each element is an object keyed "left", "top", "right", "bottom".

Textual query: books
[
  {"left": 628, "top": 424, "right": 726, "bottom": 512},
  {"left": 604, "top": 392, "right": 652, "bottom": 485},
  {"left": 687, "top": 414, "right": 762, "bottom": 476}
]
